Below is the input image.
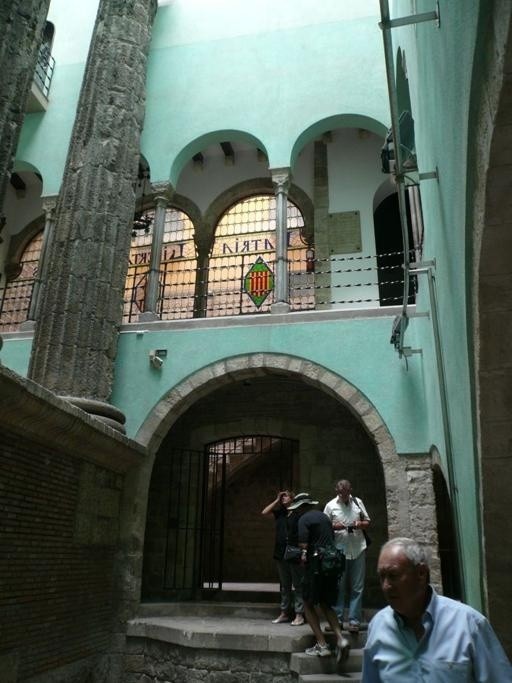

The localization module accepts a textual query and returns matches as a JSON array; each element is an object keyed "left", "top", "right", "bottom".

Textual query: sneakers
[
  {"left": 336, "top": 638, "right": 351, "bottom": 664},
  {"left": 348, "top": 624, "right": 360, "bottom": 632},
  {"left": 305, "top": 641, "right": 332, "bottom": 657},
  {"left": 325, "top": 623, "right": 343, "bottom": 631}
]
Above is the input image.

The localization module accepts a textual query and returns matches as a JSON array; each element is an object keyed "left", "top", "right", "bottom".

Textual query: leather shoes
[
  {"left": 291, "top": 618, "right": 304, "bottom": 626},
  {"left": 272, "top": 616, "right": 288, "bottom": 624}
]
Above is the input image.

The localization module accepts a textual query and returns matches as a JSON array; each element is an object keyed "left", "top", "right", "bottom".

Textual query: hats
[{"left": 286, "top": 492, "right": 319, "bottom": 510}]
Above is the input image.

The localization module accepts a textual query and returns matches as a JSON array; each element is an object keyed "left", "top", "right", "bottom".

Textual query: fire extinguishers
[{"left": 299, "top": 227, "right": 315, "bottom": 272}]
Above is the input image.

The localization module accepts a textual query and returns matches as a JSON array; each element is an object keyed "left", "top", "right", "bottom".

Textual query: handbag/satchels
[{"left": 284, "top": 544, "right": 303, "bottom": 563}]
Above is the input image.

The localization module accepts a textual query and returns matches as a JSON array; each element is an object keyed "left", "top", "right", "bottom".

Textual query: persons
[
  {"left": 323, "top": 478, "right": 371, "bottom": 632},
  {"left": 361, "top": 535, "right": 511, "bottom": 682},
  {"left": 260, "top": 488, "right": 307, "bottom": 626},
  {"left": 289, "top": 491, "right": 350, "bottom": 664}
]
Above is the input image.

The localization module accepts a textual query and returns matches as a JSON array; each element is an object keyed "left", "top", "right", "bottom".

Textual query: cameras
[{"left": 347, "top": 525, "right": 357, "bottom": 533}]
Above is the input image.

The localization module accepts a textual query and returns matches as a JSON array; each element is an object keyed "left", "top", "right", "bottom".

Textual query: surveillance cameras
[{"left": 153, "top": 357, "right": 163, "bottom": 368}]
